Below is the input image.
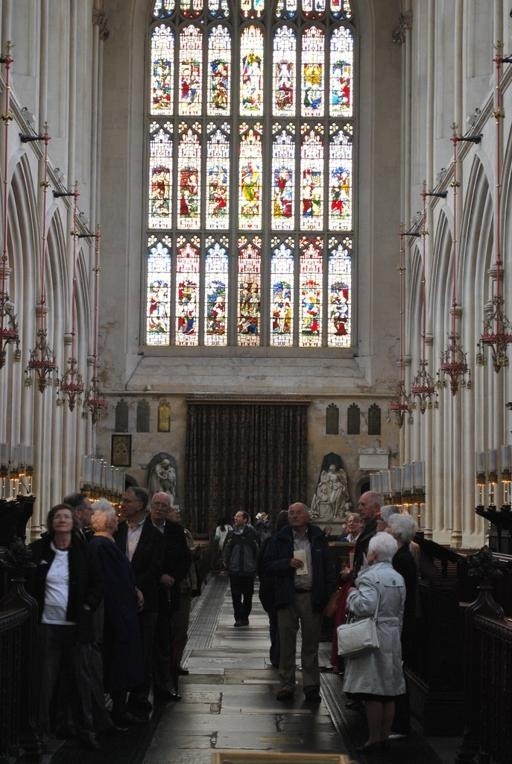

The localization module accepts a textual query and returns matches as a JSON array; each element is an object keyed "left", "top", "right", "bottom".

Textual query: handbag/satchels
[{"left": 336, "top": 588, "right": 379, "bottom": 656}]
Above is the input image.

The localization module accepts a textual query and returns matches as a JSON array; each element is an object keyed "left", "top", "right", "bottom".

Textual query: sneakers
[
  {"left": 277, "top": 689, "right": 292, "bottom": 699},
  {"left": 179, "top": 667, "right": 188, "bottom": 676},
  {"left": 233, "top": 619, "right": 250, "bottom": 626},
  {"left": 164, "top": 691, "right": 181, "bottom": 699},
  {"left": 109, "top": 714, "right": 148, "bottom": 735},
  {"left": 306, "top": 691, "right": 322, "bottom": 704},
  {"left": 83, "top": 739, "right": 100, "bottom": 749},
  {"left": 356, "top": 741, "right": 391, "bottom": 755}
]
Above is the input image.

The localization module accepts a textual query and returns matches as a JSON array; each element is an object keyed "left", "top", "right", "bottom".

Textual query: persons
[
  {"left": 215, "top": 490, "right": 421, "bottom": 758},
  {"left": 21, "top": 487, "right": 204, "bottom": 750},
  {"left": 317, "top": 464, "right": 347, "bottom": 520},
  {"left": 150, "top": 459, "right": 177, "bottom": 499}
]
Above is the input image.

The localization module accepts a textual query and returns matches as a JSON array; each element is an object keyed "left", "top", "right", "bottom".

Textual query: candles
[{"left": 1, "top": 472, "right": 31, "bottom": 501}]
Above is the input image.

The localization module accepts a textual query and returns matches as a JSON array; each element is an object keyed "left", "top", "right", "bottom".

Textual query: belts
[{"left": 296, "top": 589, "right": 311, "bottom": 593}]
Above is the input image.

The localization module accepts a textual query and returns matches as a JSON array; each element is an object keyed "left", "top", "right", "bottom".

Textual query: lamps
[
  {"left": 435, "top": 121, "right": 483, "bottom": 397},
  {"left": 0, "top": 38, "right": 25, "bottom": 371},
  {"left": 19, "top": 120, "right": 58, "bottom": 391},
  {"left": 476, "top": 38, "right": 512, "bottom": 374},
  {"left": 407, "top": 178, "right": 448, "bottom": 415},
  {"left": 389, "top": 222, "right": 420, "bottom": 429},
  {"left": 80, "top": 222, "right": 107, "bottom": 425},
  {"left": 52, "top": 180, "right": 85, "bottom": 411}
]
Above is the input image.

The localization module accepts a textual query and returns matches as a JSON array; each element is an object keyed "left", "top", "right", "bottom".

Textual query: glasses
[
  {"left": 153, "top": 502, "right": 168, "bottom": 508},
  {"left": 120, "top": 499, "right": 129, "bottom": 505}
]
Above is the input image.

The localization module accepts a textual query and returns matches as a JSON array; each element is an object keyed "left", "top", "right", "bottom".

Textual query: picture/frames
[{"left": 111, "top": 433, "right": 132, "bottom": 467}]
